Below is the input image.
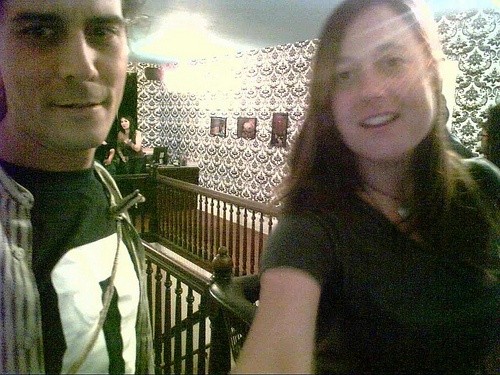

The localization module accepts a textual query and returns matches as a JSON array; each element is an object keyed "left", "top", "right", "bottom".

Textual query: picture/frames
[
  {"left": 209, "top": 116, "right": 227, "bottom": 137},
  {"left": 271, "top": 111, "right": 288, "bottom": 148},
  {"left": 237, "top": 117, "right": 257, "bottom": 139}
]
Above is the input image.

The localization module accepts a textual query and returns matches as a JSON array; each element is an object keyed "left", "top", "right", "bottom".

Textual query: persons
[
  {"left": 94, "top": 140, "right": 116, "bottom": 177},
  {"left": 0, "top": 0, "right": 156, "bottom": 375},
  {"left": 117, "top": 114, "right": 146, "bottom": 199},
  {"left": 227, "top": 1, "right": 500, "bottom": 375}
]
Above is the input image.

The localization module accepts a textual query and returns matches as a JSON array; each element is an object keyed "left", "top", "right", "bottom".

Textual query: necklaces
[{"left": 366, "top": 176, "right": 420, "bottom": 221}]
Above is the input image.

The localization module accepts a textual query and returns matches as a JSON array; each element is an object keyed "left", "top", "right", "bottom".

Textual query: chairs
[{"left": 128, "top": 145, "right": 170, "bottom": 209}]
